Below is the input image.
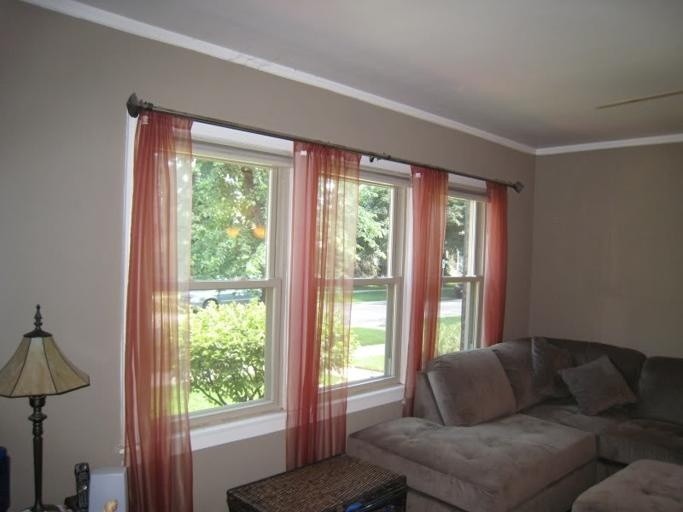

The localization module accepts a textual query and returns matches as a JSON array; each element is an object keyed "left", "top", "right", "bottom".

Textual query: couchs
[{"left": 346, "top": 373, "right": 683, "bottom": 511}]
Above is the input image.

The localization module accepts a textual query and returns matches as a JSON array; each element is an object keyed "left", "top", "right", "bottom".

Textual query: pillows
[{"left": 426, "top": 337, "right": 683, "bottom": 425}]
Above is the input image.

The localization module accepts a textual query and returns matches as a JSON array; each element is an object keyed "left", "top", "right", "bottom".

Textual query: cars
[{"left": 178, "top": 286, "right": 263, "bottom": 311}]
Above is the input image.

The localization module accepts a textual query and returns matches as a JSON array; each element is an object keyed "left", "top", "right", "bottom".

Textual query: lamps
[{"left": 0, "top": 304, "right": 91, "bottom": 512}]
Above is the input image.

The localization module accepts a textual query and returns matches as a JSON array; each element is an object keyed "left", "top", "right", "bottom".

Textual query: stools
[{"left": 570, "top": 457, "right": 683, "bottom": 512}]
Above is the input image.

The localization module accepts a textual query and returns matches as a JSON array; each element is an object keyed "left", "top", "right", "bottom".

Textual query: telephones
[{"left": 64, "top": 463, "right": 90, "bottom": 512}]
